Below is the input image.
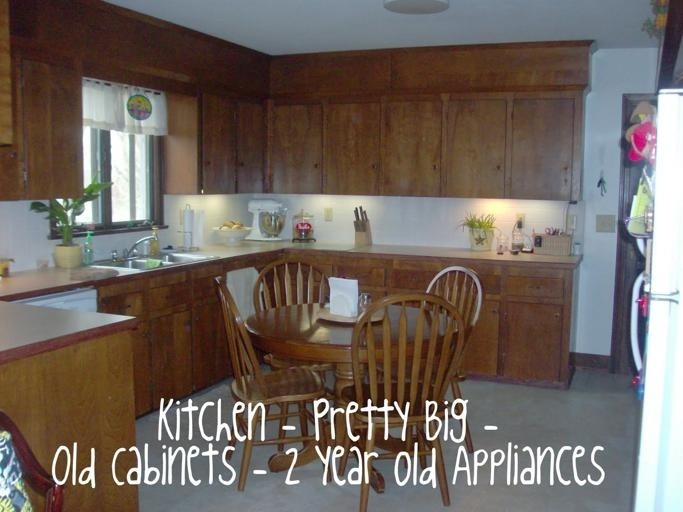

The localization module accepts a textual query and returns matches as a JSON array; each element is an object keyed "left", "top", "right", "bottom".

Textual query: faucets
[{"left": 118, "top": 234, "right": 159, "bottom": 259}]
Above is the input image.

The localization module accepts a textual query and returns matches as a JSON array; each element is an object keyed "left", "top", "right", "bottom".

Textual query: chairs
[{"left": 212, "top": 255, "right": 485, "bottom": 512}]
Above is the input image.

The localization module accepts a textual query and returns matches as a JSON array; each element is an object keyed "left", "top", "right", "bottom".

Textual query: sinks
[
  {"left": 91, "top": 257, "right": 168, "bottom": 271},
  {"left": 134, "top": 251, "right": 193, "bottom": 264}
]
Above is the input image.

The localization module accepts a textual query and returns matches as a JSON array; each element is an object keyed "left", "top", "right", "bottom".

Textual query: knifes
[{"left": 353, "top": 206, "right": 368, "bottom": 222}]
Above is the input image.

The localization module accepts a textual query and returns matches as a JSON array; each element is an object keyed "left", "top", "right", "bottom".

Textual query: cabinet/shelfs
[
  {"left": 99, "top": 268, "right": 195, "bottom": 419},
  {"left": 160, "top": 92, "right": 267, "bottom": 194},
  {"left": 16, "top": 56, "right": 82, "bottom": 201},
  {"left": 284, "top": 250, "right": 571, "bottom": 390},
  {"left": 191, "top": 248, "right": 283, "bottom": 392},
  {"left": 264, "top": 92, "right": 584, "bottom": 200}
]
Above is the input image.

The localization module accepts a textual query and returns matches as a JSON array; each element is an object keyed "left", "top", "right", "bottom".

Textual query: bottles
[
  {"left": 291, "top": 209, "right": 316, "bottom": 243},
  {"left": 151, "top": 226, "right": 159, "bottom": 254}
]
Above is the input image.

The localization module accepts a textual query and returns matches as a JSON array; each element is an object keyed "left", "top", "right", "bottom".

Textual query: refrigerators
[{"left": 632, "top": 87, "right": 683, "bottom": 512}]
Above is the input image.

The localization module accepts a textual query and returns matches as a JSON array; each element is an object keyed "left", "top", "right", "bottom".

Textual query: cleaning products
[{"left": 182, "top": 202, "right": 192, "bottom": 232}]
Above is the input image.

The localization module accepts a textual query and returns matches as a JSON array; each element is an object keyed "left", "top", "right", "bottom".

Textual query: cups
[
  {"left": 497, "top": 236, "right": 506, "bottom": 254},
  {"left": 361, "top": 293, "right": 371, "bottom": 304}
]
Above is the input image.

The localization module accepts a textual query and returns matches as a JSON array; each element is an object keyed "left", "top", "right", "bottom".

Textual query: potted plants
[
  {"left": 456, "top": 209, "right": 501, "bottom": 252},
  {"left": 28, "top": 176, "right": 115, "bottom": 271}
]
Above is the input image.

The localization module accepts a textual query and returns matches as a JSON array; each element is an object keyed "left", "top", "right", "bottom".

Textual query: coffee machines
[{"left": 244, "top": 199, "right": 288, "bottom": 240}]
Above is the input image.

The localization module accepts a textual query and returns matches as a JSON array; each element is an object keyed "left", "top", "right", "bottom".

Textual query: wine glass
[{"left": 214, "top": 226, "right": 253, "bottom": 247}]
